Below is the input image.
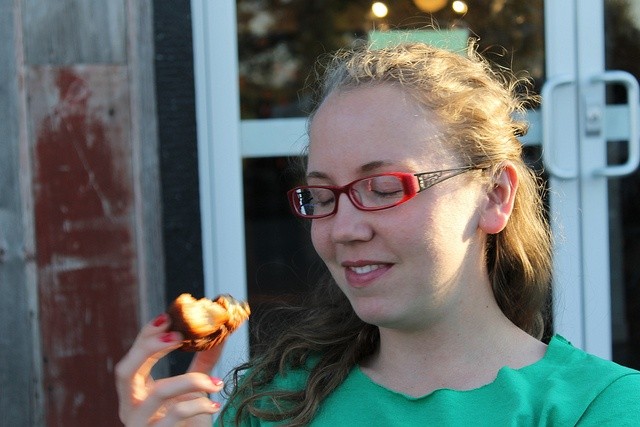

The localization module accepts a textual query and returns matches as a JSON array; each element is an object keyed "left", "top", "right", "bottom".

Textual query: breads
[{"left": 164, "top": 294, "right": 252, "bottom": 352}]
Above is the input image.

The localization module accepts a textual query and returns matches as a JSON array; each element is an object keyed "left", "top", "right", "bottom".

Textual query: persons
[{"left": 115, "top": 16, "right": 639, "bottom": 425}]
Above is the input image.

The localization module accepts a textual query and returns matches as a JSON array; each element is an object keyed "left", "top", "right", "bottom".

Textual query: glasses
[{"left": 286, "top": 166, "right": 479, "bottom": 220}]
